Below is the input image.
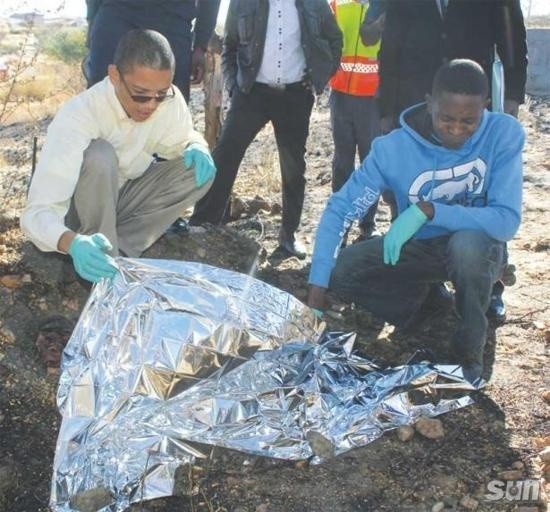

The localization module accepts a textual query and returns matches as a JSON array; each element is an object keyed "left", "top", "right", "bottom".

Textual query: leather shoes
[{"left": 485, "top": 295, "right": 505, "bottom": 319}]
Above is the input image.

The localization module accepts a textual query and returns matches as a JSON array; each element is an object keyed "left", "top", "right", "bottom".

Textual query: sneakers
[{"left": 278, "top": 237, "right": 306, "bottom": 257}]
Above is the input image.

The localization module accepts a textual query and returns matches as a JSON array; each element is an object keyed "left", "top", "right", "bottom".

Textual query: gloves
[
  {"left": 383, "top": 204, "right": 429, "bottom": 266},
  {"left": 184, "top": 143, "right": 216, "bottom": 187},
  {"left": 67, "top": 233, "right": 119, "bottom": 282},
  {"left": 309, "top": 307, "right": 323, "bottom": 318}
]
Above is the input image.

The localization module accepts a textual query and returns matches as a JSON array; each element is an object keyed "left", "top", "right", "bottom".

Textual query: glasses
[{"left": 117, "top": 68, "right": 175, "bottom": 103}]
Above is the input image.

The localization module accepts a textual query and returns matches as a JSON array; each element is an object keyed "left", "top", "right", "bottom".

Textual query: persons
[{"left": 20, "top": 0, "right": 528, "bottom": 391}]
